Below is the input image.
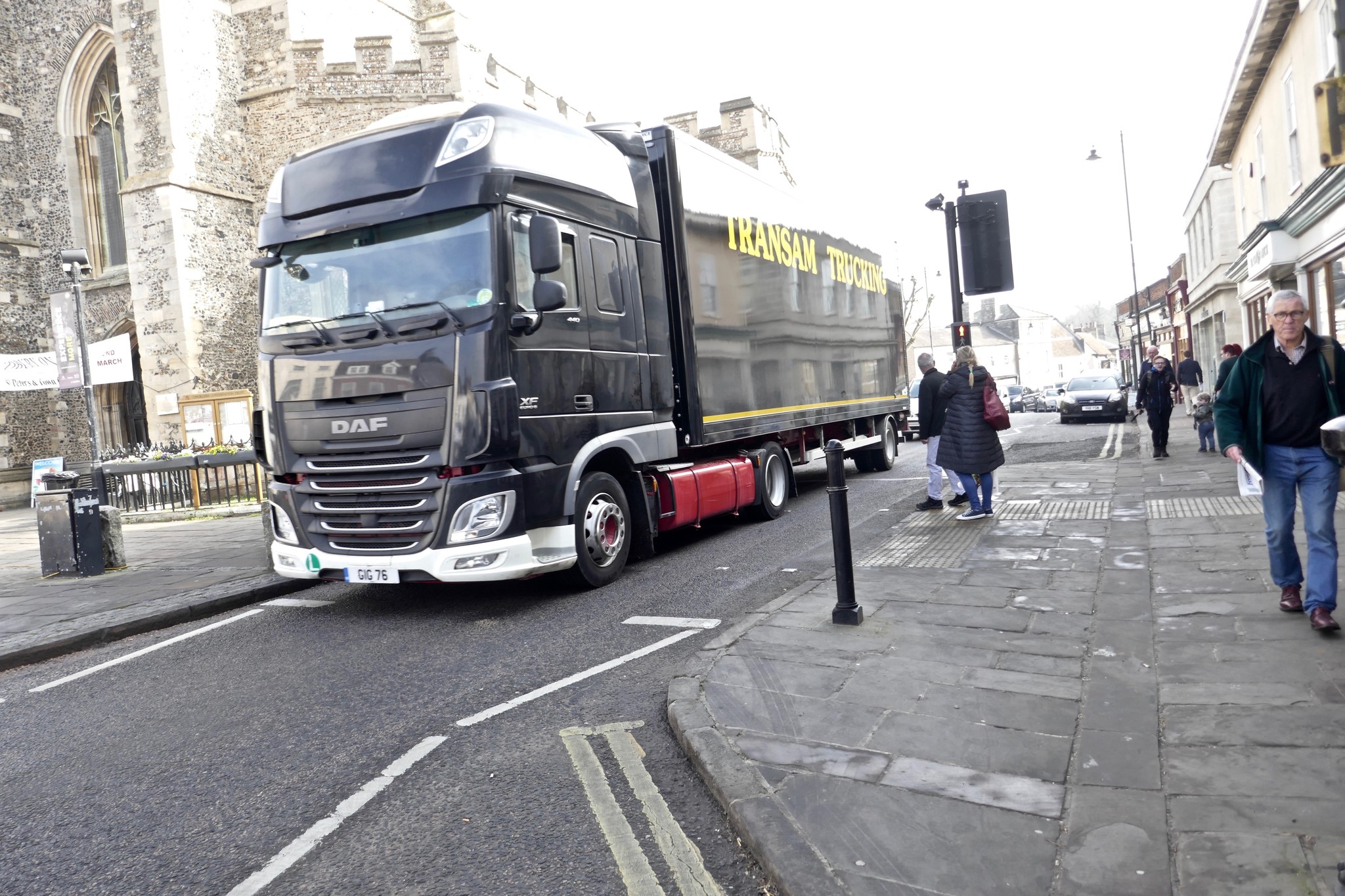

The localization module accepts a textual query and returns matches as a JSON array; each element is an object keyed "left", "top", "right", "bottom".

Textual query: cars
[
  {"left": 1057, "top": 375, "right": 1127, "bottom": 424},
  {"left": 996, "top": 368, "right": 1133, "bottom": 415}
]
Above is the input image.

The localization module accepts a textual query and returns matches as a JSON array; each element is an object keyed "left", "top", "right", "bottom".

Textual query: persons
[
  {"left": 1211, "top": 289, "right": 1345, "bottom": 631},
  {"left": 1191, "top": 393, "right": 1215, "bottom": 451},
  {"left": 935, "top": 345, "right": 1005, "bottom": 520},
  {"left": 1177, "top": 350, "right": 1203, "bottom": 416},
  {"left": 1136, "top": 355, "right": 1178, "bottom": 458},
  {"left": 1139, "top": 345, "right": 1173, "bottom": 383},
  {"left": 916, "top": 353, "right": 969, "bottom": 509},
  {"left": 1212, "top": 344, "right": 1242, "bottom": 394}
]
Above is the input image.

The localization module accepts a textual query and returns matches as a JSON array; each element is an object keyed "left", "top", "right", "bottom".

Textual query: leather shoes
[
  {"left": 1310, "top": 606, "right": 1340, "bottom": 631},
  {"left": 1279, "top": 585, "right": 1302, "bottom": 611}
]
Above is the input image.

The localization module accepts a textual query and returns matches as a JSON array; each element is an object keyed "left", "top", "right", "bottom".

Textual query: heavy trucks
[{"left": 247, "top": 102, "right": 912, "bottom": 596}]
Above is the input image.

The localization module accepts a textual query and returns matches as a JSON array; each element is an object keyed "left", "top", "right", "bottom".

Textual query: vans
[{"left": 901, "top": 375, "right": 935, "bottom": 440}]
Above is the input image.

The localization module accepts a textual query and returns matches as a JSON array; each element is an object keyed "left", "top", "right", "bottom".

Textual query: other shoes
[
  {"left": 1193, "top": 405, "right": 1197, "bottom": 409},
  {"left": 1209, "top": 447, "right": 1215, "bottom": 451},
  {"left": 1187, "top": 414, "right": 1191, "bottom": 416},
  {"left": 1198, "top": 447, "right": 1206, "bottom": 452},
  {"left": 1161, "top": 448, "right": 1169, "bottom": 456},
  {"left": 1152, "top": 447, "right": 1161, "bottom": 457}
]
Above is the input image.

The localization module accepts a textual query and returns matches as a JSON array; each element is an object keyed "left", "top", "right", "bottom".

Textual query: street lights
[
  {"left": 60, "top": 247, "right": 100, "bottom": 463},
  {"left": 1088, "top": 130, "right": 1143, "bottom": 361}
]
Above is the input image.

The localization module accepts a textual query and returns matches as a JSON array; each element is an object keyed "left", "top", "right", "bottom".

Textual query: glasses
[
  {"left": 1154, "top": 362, "right": 1165, "bottom": 365},
  {"left": 1269, "top": 310, "right": 1305, "bottom": 321},
  {"left": 1220, "top": 353, "right": 1227, "bottom": 357}
]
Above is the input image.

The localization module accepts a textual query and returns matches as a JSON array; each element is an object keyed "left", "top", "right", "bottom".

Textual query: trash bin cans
[
  {"left": 41, "top": 470, "right": 79, "bottom": 489},
  {"left": 35, "top": 487, "right": 104, "bottom": 578}
]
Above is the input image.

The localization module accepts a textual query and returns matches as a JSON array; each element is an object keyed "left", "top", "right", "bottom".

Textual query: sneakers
[
  {"left": 955, "top": 508, "right": 985, "bottom": 520},
  {"left": 947, "top": 492, "right": 969, "bottom": 506},
  {"left": 916, "top": 496, "right": 943, "bottom": 510},
  {"left": 985, "top": 509, "right": 993, "bottom": 517}
]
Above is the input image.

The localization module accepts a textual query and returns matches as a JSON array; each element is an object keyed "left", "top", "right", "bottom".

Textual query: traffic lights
[{"left": 951, "top": 322, "right": 972, "bottom": 352}]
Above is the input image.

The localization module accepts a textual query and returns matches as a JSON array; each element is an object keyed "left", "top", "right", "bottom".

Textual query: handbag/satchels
[
  {"left": 1337, "top": 466, "right": 1345, "bottom": 492},
  {"left": 972, "top": 468, "right": 998, "bottom": 495},
  {"left": 982, "top": 373, "right": 1011, "bottom": 432}
]
